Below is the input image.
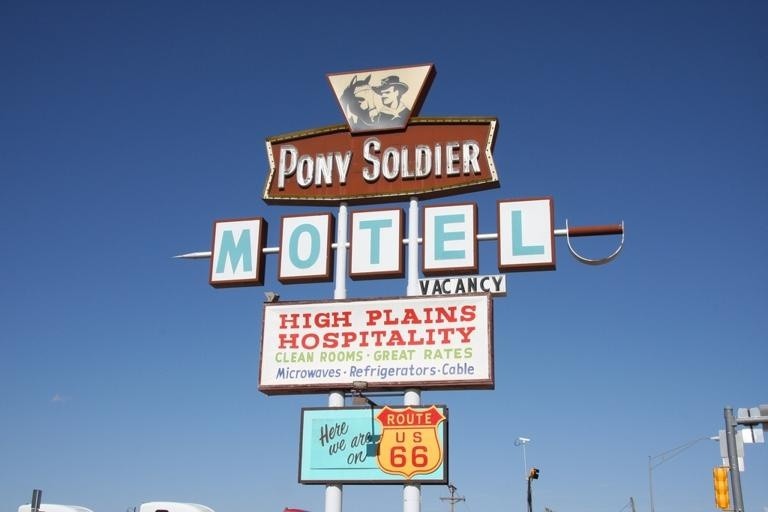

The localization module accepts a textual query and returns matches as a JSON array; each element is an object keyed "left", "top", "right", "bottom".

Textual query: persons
[{"left": 359, "top": 74, "right": 410, "bottom": 128}]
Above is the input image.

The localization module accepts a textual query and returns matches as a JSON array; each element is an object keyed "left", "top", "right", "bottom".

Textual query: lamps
[{"left": 353, "top": 382, "right": 368, "bottom": 403}]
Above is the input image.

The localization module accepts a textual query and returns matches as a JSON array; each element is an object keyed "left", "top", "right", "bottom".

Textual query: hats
[{"left": 371, "top": 77, "right": 408, "bottom": 95}]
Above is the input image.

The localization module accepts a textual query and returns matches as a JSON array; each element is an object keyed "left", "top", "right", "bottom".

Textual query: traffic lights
[{"left": 713, "top": 467, "right": 729, "bottom": 509}]
